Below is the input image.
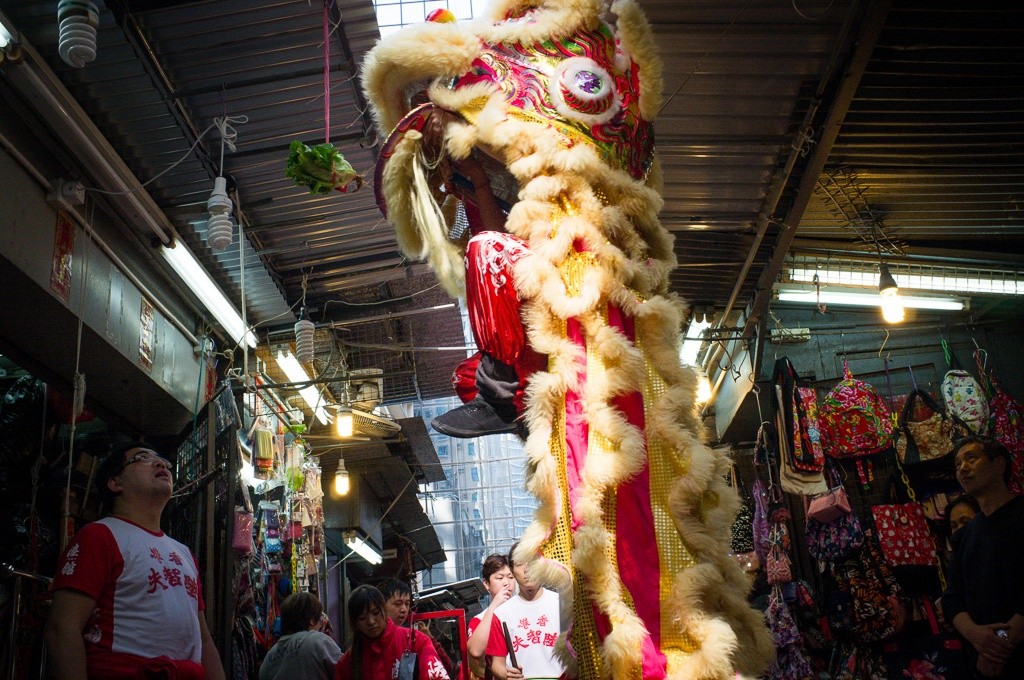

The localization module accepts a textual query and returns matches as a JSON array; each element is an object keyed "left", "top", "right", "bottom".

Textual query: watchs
[{"left": 995, "top": 629, "right": 1008, "bottom": 640}]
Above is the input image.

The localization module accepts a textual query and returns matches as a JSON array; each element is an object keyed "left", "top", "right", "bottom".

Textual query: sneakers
[{"left": 431, "top": 398, "right": 516, "bottom": 438}]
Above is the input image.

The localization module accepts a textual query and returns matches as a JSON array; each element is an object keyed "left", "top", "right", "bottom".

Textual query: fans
[{"left": 323, "top": 367, "right": 402, "bottom": 437}]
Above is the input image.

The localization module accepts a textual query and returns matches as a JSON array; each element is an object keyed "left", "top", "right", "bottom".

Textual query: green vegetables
[{"left": 284, "top": 140, "right": 357, "bottom": 196}]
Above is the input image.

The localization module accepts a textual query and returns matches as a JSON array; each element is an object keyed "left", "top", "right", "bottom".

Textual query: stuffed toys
[{"left": 361, "top": 0, "right": 774, "bottom": 680}]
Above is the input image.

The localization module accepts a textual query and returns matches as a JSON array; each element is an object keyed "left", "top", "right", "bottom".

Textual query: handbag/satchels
[{"left": 725, "top": 356, "right": 1024, "bottom": 680}]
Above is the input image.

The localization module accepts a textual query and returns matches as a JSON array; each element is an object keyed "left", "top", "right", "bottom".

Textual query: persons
[
  {"left": 467, "top": 553, "right": 516, "bottom": 680},
  {"left": 332, "top": 584, "right": 452, "bottom": 680},
  {"left": 377, "top": 576, "right": 411, "bottom": 626},
  {"left": 430, "top": 155, "right": 547, "bottom": 441},
  {"left": 47, "top": 441, "right": 226, "bottom": 680},
  {"left": 484, "top": 542, "right": 568, "bottom": 680},
  {"left": 261, "top": 592, "right": 345, "bottom": 680},
  {"left": 933, "top": 437, "right": 1024, "bottom": 680}
]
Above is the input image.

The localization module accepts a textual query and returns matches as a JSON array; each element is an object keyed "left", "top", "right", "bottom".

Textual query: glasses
[{"left": 113, "top": 452, "right": 172, "bottom": 478}]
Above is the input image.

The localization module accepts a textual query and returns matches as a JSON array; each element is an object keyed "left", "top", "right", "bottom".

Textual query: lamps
[
  {"left": 772, "top": 282, "right": 972, "bottom": 312},
  {"left": 269, "top": 342, "right": 331, "bottom": 426},
  {"left": 0, "top": 13, "right": 259, "bottom": 350},
  {"left": 442, "top": 602, "right": 455, "bottom": 610},
  {"left": 343, "top": 530, "right": 383, "bottom": 565}
]
